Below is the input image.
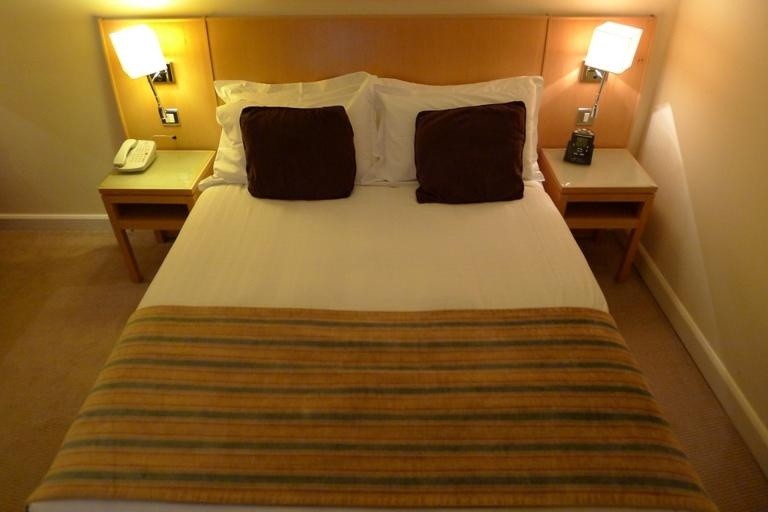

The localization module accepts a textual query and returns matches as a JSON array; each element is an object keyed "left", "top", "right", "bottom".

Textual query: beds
[{"left": 27, "top": 15, "right": 719, "bottom": 512}]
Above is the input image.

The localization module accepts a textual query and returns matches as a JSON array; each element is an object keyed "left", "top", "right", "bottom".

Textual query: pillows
[
  {"left": 199, "top": 71, "right": 369, "bottom": 190},
  {"left": 370, "top": 75, "right": 543, "bottom": 189},
  {"left": 413, "top": 100, "right": 527, "bottom": 203},
  {"left": 237, "top": 105, "right": 357, "bottom": 200}
]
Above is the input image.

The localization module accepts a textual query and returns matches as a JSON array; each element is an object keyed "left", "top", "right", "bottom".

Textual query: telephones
[{"left": 112, "top": 139, "right": 156, "bottom": 173}]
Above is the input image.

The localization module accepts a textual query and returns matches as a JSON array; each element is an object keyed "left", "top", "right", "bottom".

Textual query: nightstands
[
  {"left": 539, "top": 147, "right": 659, "bottom": 285},
  {"left": 98, "top": 150, "right": 219, "bottom": 285}
]
